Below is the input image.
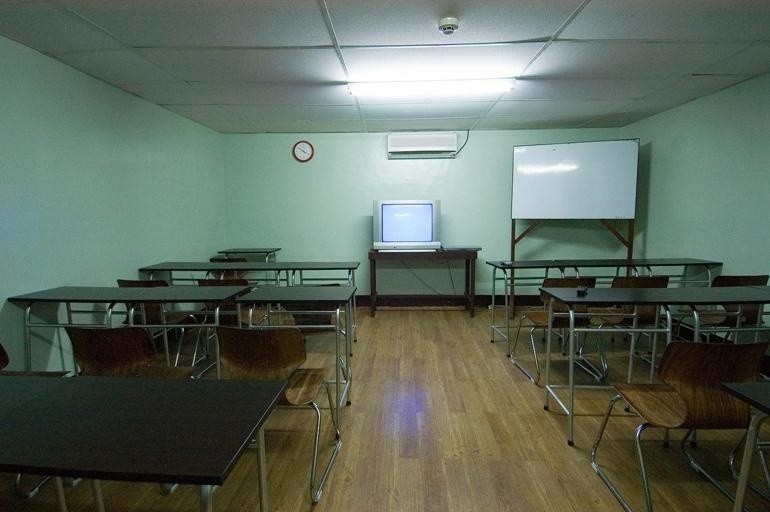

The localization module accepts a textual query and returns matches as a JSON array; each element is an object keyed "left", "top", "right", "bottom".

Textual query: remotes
[{"left": 575, "top": 285, "right": 588, "bottom": 295}]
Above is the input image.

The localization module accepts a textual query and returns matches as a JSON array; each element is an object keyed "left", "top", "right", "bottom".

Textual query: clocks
[{"left": 293, "top": 141, "right": 314, "bottom": 162}]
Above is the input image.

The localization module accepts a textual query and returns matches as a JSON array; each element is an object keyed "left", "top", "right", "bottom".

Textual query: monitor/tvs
[{"left": 372, "top": 198, "right": 441, "bottom": 250}]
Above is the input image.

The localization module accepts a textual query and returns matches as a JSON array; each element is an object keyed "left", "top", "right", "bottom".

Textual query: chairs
[
  {"left": 589, "top": 340, "right": 769, "bottom": 511},
  {"left": 2, "top": 248, "right": 374, "bottom": 512},
  {"left": 482, "top": 257, "right": 770, "bottom": 451}
]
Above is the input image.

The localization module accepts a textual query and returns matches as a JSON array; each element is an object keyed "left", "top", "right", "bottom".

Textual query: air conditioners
[{"left": 386, "top": 131, "right": 458, "bottom": 160}]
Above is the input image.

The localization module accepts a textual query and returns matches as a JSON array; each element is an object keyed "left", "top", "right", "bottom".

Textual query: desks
[{"left": 368, "top": 247, "right": 482, "bottom": 319}]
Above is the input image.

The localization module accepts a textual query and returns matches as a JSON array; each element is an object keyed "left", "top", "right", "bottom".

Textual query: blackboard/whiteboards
[{"left": 511, "top": 138, "right": 640, "bottom": 220}]
